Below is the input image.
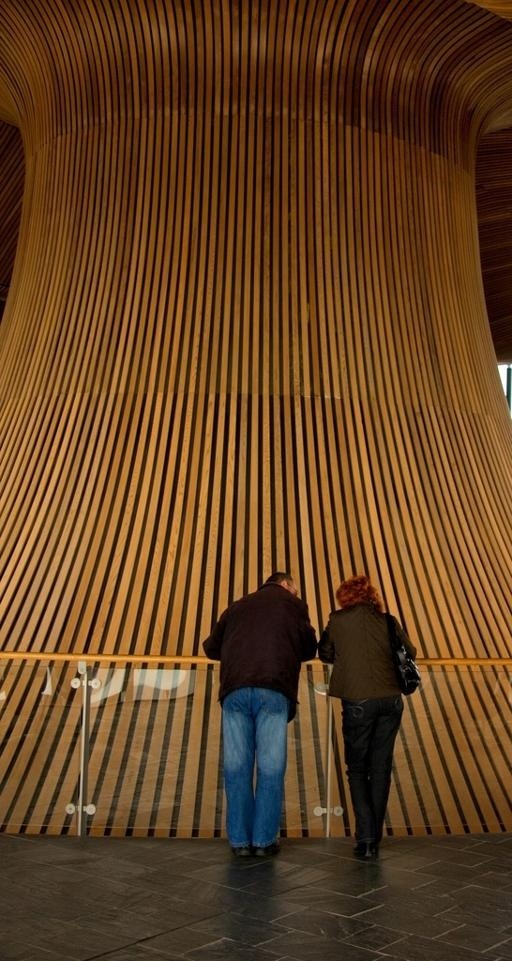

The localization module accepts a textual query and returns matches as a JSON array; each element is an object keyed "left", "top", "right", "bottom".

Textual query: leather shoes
[
  {"left": 353, "top": 841, "right": 379, "bottom": 854},
  {"left": 361, "top": 840, "right": 374, "bottom": 858}
]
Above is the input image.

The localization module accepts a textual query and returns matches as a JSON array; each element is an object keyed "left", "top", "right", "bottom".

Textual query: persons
[
  {"left": 201, "top": 573, "right": 318, "bottom": 858},
  {"left": 317, "top": 577, "right": 417, "bottom": 858}
]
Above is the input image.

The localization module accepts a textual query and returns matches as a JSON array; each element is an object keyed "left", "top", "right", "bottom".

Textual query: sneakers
[
  {"left": 231, "top": 845, "right": 250, "bottom": 856},
  {"left": 255, "top": 838, "right": 281, "bottom": 857}
]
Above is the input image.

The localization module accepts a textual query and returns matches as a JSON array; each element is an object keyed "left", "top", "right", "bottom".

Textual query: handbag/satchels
[{"left": 384, "top": 611, "right": 421, "bottom": 695}]
